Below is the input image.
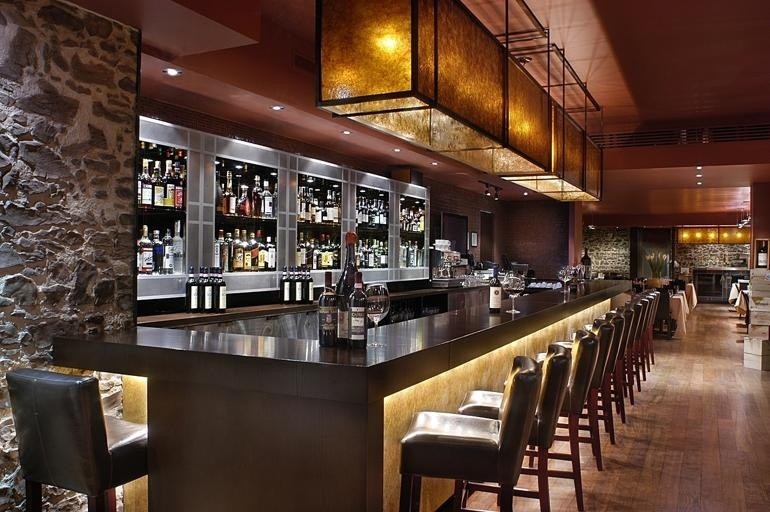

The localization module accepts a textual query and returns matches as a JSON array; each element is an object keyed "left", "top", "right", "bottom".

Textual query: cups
[
  {"left": 528, "top": 281, "right": 562, "bottom": 289},
  {"left": 462, "top": 274, "right": 485, "bottom": 286}
]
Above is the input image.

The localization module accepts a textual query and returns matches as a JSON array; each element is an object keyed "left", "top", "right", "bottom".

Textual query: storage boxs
[{"left": 744, "top": 268, "right": 770, "bottom": 371}]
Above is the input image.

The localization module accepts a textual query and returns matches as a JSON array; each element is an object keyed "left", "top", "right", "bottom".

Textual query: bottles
[
  {"left": 319, "top": 272, "right": 337, "bottom": 346},
  {"left": 336, "top": 232, "right": 365, "bottom": 350},
  {"left": 758, "top": 240, "right": 767, "bottom": 267},
  {"left": 200, "top": 266, "right": 226, "bottom": 312},
  {"left": 401, "top": 239, "right": 423, "bottom": 266},
  {"left": 291, "top": 266, "right": 314, "bottom": 303},
  {"left": 281, "top": 266, "right": 290, "bottom": 304},
  {"left": 138, "top": 221, "right": 184, "bottom": 273},
  {"left": 298, "top": 184, "right": 342, "bottom": 227},
  {"left": 215, "top": 226, "right": 276, "bottom": 270},
  {"left": 186, "top": 267, "right": 199, "bottom": 313},
  {"left": 217, "top": 169, "right": 277, "bottom": 220},
  {"left": 399, "top": 205, "right": 423, "bottom": 232},
  {"left": 356, "top": 238, "right": 388, "bottom": 268},
  {"left": 489, "top": 264, "right": 501, "bottom": 314},
  {"left": 348, "top": 272, "right": 367, "bottom": 350},
  {"left": 298, "top": 231, "right": 340, "bottom": 269},
  {"left": 356, "top": 195, "right": 388, "bottom": 231},
  {"left": 139, "top": 157, "right": 183, "bottom": 208}
]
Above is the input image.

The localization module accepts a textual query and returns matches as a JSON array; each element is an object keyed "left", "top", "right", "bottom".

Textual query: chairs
[{"left": 7, "top": 367, "right": 148, "bottom": 512}]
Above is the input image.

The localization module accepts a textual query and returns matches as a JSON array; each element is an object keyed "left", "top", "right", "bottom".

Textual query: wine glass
[
  {"left": 559, "top": 265, "right": 586, "bottom": 294},
  {"left": 364, "top": 283, "right": 391, "bottom": 349},
  {"left": 502, "top": 270, "right": 524, "bottom": 314},
  {"left": 432, "top": 257, "right": 456, "bottom": 280}
]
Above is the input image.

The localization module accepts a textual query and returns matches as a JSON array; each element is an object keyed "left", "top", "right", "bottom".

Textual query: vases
[{"left": 645, "top": 277, "right": 661, "bottom": 287}]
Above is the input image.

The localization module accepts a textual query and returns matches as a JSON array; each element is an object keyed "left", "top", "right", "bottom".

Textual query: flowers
[{"left": 645, "top": 252, "right": 672, "bottom": 278}]
